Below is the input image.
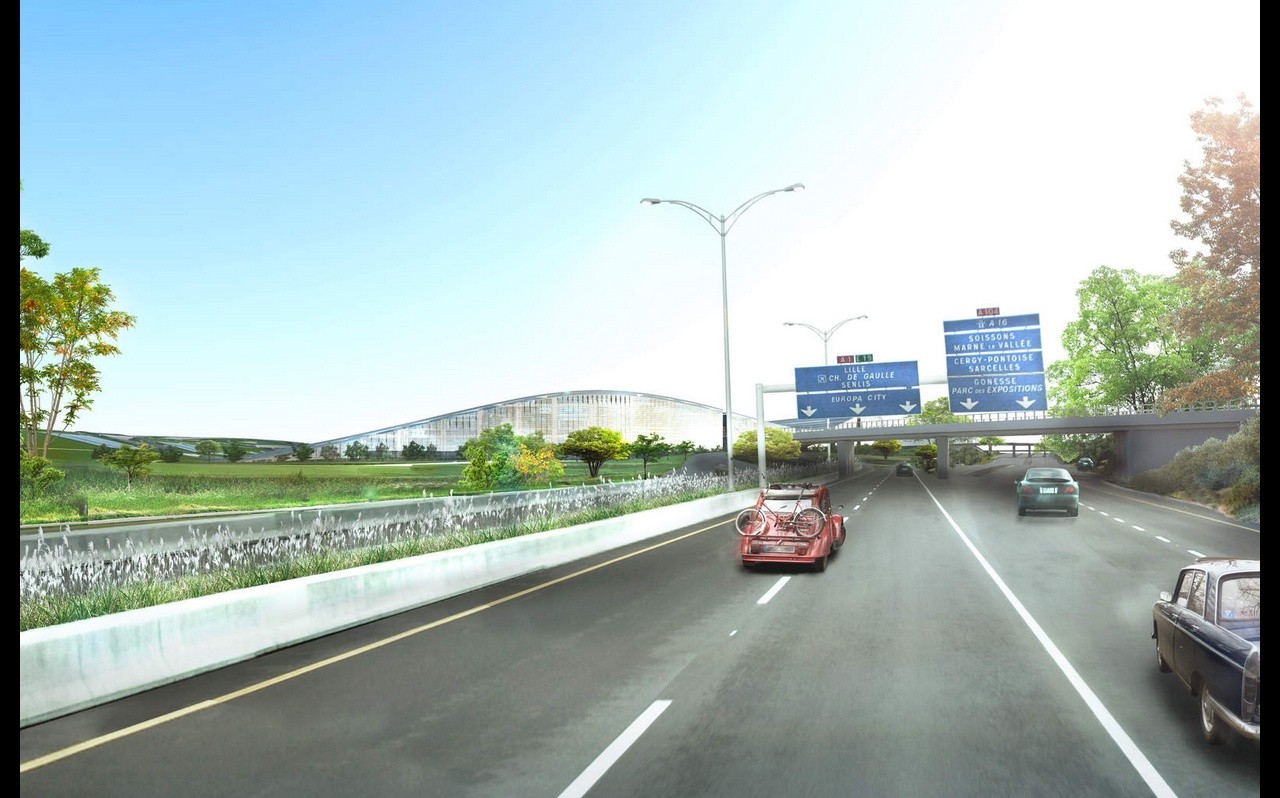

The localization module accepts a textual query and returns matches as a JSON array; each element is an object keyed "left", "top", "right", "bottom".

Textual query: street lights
[
  {"left": 640, "top": 182, "right": 806, "bottom": 493},
  {"left": 783, "top": 316, "right": 868, "bottom": 463}
]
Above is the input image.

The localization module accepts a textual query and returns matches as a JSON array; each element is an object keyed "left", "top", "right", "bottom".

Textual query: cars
[
  {"left": 1077, "top": 456, "right": 1094, "bottom": 471},
  {"left": 1013, "top": 467, "right": 1078, "bottom": 516},
  {"left": 894, "top": 461, "right": 914, "bottom": 476},
  {"left": 1151, "top": 555, "right": 1261, "bottom": 746},
  {"left": 739, "top": 484, "right": 846, "bottom": 573}
]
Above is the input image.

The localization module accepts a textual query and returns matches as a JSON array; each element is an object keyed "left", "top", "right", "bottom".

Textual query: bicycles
[{"left": 734, "top": 472, "right": 829, "bottom": 538}]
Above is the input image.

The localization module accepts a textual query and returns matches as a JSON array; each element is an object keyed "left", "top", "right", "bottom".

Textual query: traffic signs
[
  {"left": 943, "top": 375, "right": 1047, "bottom": 413},
  {"left": 794, "top": 388, "right": 921, "bottom": 419}
]
